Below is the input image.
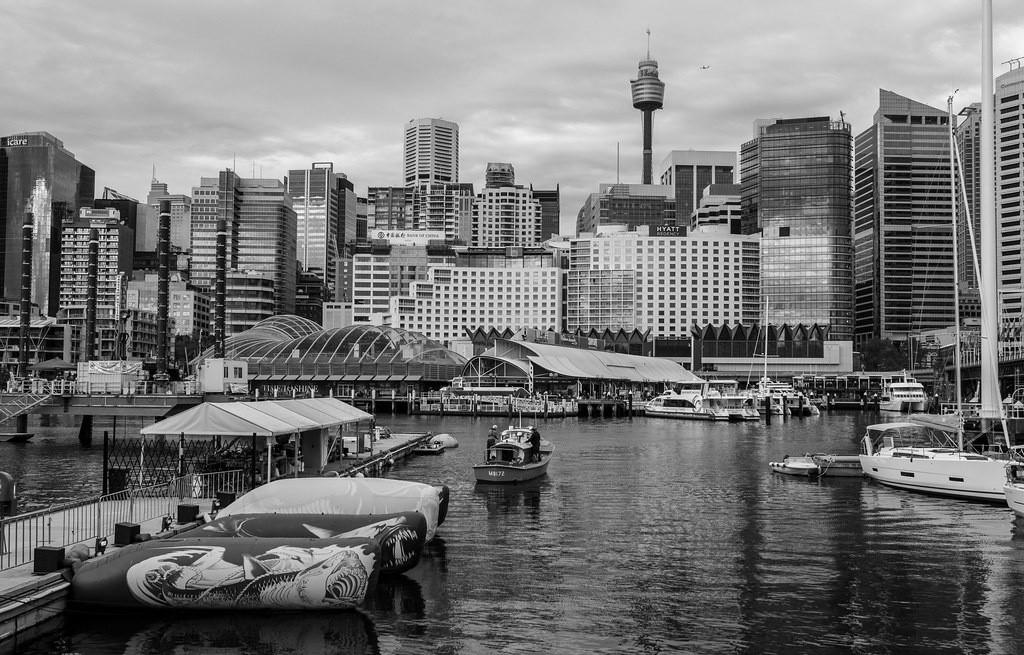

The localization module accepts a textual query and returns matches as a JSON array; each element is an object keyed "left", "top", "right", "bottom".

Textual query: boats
[
  {"left": 637, "top": 361, "right": 929, "bottom": 423},
  {"left": 413, "top": 441, "right": 444, "bottom": 455},
  {"left": 1004, "top": 448, "right": 1024, "bottom": 517},
  {"left": 769, "top": 452, "right": 867, "bottom": 478},
  {"left": 471, "top": 411, "right": 557, "bottom": 484}
]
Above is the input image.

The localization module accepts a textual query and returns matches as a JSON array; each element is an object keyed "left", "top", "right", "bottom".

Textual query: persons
[
  {"left": 523, "top": 428, "right": 541, "bottom": 462},
  {"left": 486, "top": 425, "right": 499, "bottom": 460}
]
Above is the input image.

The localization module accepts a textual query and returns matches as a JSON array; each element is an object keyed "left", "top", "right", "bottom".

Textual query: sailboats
[{"left": 868, "top": 100, "right": 1024, "bottom": 498}]
